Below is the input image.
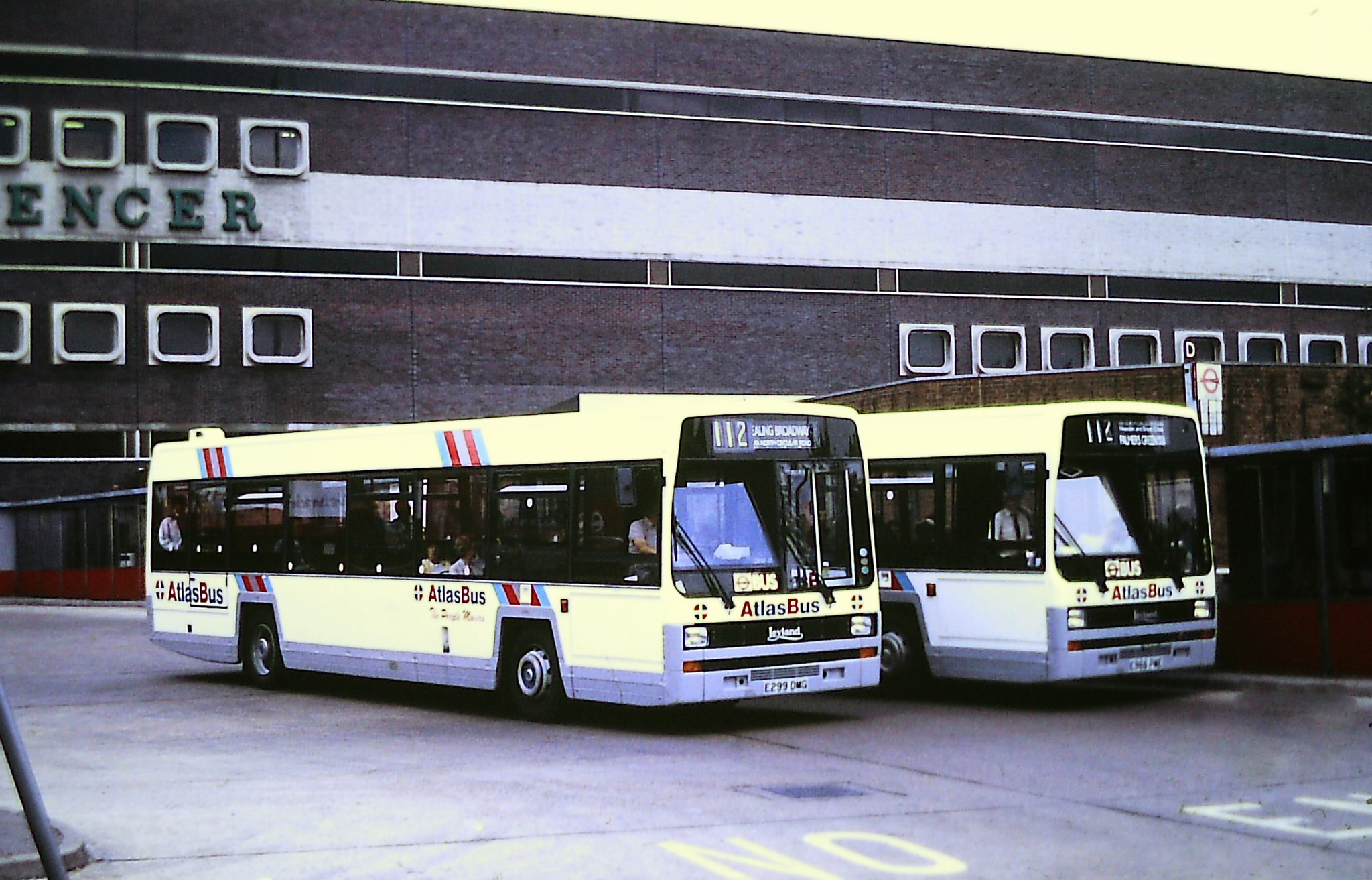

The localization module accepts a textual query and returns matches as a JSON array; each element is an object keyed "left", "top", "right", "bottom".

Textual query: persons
[
  {"left": 800, "top": 517, "right": 947, "bottom": 569},
  {"left": 987, "top": 486, "right": 1033, "bottom": 570},
  {"left": 158, "top": 491, "right": 659, "bottom": 586}
]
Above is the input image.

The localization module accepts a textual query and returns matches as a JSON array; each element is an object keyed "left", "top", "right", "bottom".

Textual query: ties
[{"left": 1011, "top": 513, "right": 1021, "bottom": 539}]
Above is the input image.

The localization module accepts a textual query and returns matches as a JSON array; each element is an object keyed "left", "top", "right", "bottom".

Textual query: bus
[
  {"left": 145, "top": 391, "right": 883, "bottom": 724},
  {"left": 777, "top": 400, "right": 1216, "bottom": 700}
]
